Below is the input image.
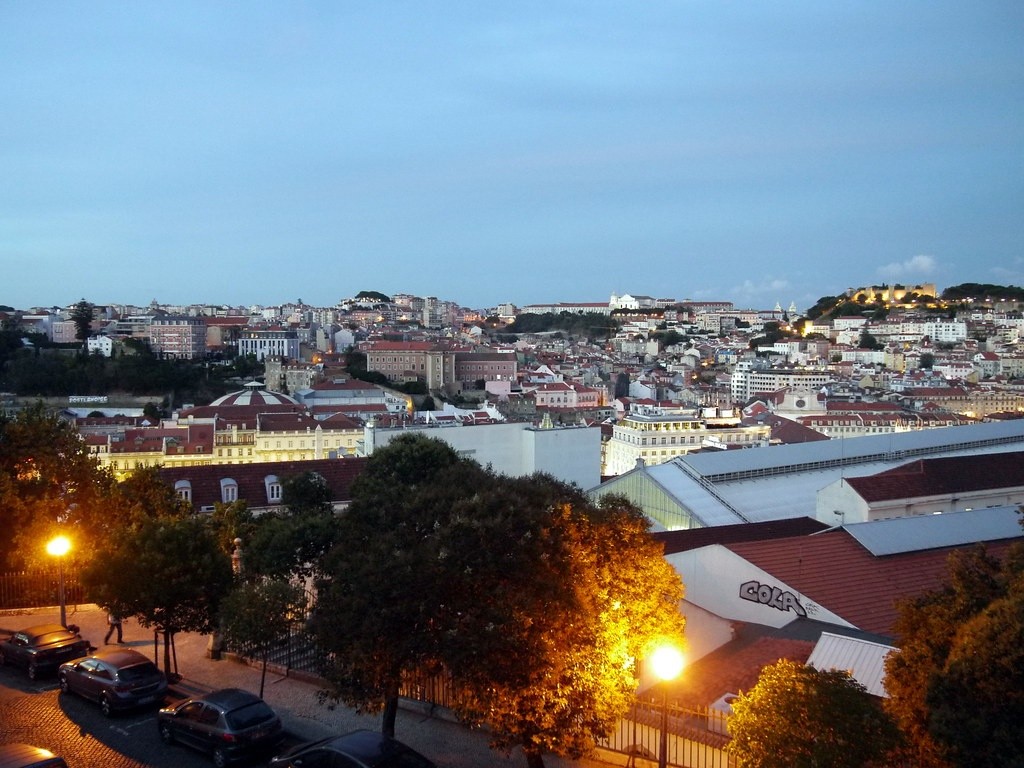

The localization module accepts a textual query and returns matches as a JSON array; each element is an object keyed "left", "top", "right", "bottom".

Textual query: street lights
[
  {"left": 656, "top": 651, "right": 679, "bottom": 768},
  {"left": 51, "top": 540, "right": 69, "bottom": 627}
]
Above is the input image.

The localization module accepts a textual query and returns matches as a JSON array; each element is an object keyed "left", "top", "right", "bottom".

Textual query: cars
[
  {"left": 0, "top": 743, "right": 68, "bottom": 768},
  {"left": 268, "top": 729, "right": 438, "bottom": 768},
  {"left": 158, "top": 688, "right": 282, "bottom": 768},
  {"left": 58, "top": 649, "right": 168, "bottom": 719},
  {"left": 0, "top": 624, "right": 87, "bottom": 683}
]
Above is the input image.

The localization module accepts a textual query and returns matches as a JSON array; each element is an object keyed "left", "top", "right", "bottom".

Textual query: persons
[{"left": 102, "top": 597, "right": 126, "bottom": 646}]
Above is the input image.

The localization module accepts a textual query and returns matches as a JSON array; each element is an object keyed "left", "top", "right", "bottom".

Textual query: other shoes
[
  {"left": 104, "top": 639, "right": 108, "bottom": 645},
  {"left": 89, "top": 647, "right": 97, "bottom": 651},
  {"left": 118, "top": 640, "right": 124, "bottom": 643}
]
